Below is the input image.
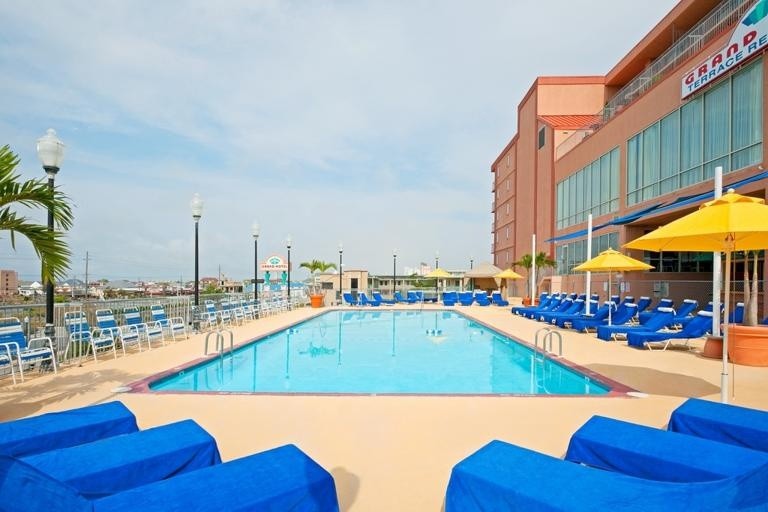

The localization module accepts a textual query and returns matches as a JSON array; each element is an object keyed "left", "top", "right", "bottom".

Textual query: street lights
[
  {"left": 391, "top": 310, "right": 396, "bottom": 361},
  {"left": 470, "top": 253, "right": 475, "bottom": 295},
  {"left": 286, "top": 235, "right": 293, "bottom": 310},
  {"left": 34, "top": 128, "right": 68, "bottom": 374},
  {"left": 435, "top": 251, "right": 440, "bottom": 304},
  {"left": 392, "top": 248, "right": 396, "bottom": 303},
  {"left": 336, "top": 311, "right": 343, "bottom": 373},
  {"left": 284, "top": 331, "right": 291, "bottom": 389},
  {"left": 338, "top": 242, "right": 343, "bottom": 305},
  {"left": 251, "top": 221, "right": 261, "bottom": 321},
  {"left": 190, "top": 192, "right": 205, "bottom": 336}
]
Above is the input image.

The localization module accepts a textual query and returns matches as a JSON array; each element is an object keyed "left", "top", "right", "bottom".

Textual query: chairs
[
  {"left": 0, "top": 317, "right": 57, "bottom": 387},
  {"left": 512, "top": 292, "right": 744, "bottom": 353},
  {"left": 190, "top": 295, "right": 259, "bottom": 333},
  {"left": 63, "top": 304, "right": 189, "bottom": 362},
  {"left": 442, "top": 289, "right": 509, "bottom": 306},
  {"left": 342, "top": 291, "right": 437, "bottom": 307}
]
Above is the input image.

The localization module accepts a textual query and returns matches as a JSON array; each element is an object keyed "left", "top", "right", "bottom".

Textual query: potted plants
[
  {"left": 512, "top": 251, "right": 557, "bottom": 306},
  {"left": 300, "top": 260, "right": 338, "bottom": 307}
]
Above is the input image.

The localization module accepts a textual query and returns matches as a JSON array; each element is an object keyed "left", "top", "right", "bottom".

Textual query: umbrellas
[
  {"left": 491, "top": 268, "right": 525, "bottom": 305},
  {"left": 425, "top": 310, "right": 448, "bottom": 345},
  {"left": 571, "top": 247, "right": 656, "bottom": 326},
  {"left": 426, "top": 268, "right": 451, "bottom": 300},
  {"left": 466, "top": 262, "right": 504, "bottom": 278},
  {"left": 620, "top": 189, "right": 768, "bottom": 404}
]
